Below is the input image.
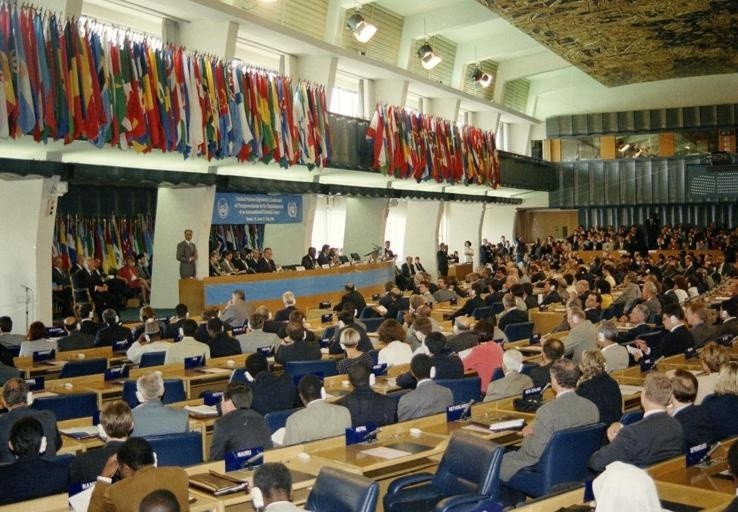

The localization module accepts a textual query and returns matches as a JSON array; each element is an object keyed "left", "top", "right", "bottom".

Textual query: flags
[
  {"left": 210, "top": 224, "right": 265, "bottom": 252},
  {"left": 367, "top": 102, "right": 503, "bottom": 187},
  {"left": 2, "top": 2, "right": 334, "bottom": 171},
  {"left": 53, "top": 212, "right": 154, "bottom": 274}
]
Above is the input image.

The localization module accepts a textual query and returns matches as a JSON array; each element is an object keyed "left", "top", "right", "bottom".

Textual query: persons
[
  {"left": 1, "top": 248, "right": 321, "bottom": 410},
  {"left": 251, "top": 463, "right": 313, "bottom": 512},
  {"left": 139, "top": 489, "right": 180, "bottom": 512},
  {"left": 668, "top": 366, "right": 719, "bottom": 454},
  {"left": 67, "top": 400, "right": 136, "bottom": 494},
  {"left": 718, "top": 439, "right": 738, "bottom": 511},
  {"left": 583, "top": 371, "right": 687, "bottom": 510},
  {"left": 1, "top": 416, "right": 70, "bottom": 505},
  {"left": 282, "top": 375, "right": 353, "bottom": 447},
  {"left": 129, "top": 369, "right": 191, "bottom": 438},
  {"left": 210, "top": 380, "right": 274, "bottom": 462},
  {"left": 301, "top": 208, "right": 737, "bottom": 437},
  {"left": 0, "top": 377, "right": 64, "bottom": 464},
  {"left": 497, "top": 358, "right": 601, "bottom": 512},
  {"left": 88, "top": 437, "right": 190, "bottom": 512},
  {"left": 176, "top": 230, "right": 198, "bottom": 279}
]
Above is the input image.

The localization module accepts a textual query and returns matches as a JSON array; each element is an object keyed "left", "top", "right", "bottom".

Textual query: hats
[{"left": 145, "top": 321, "right": 160, "bottom": 334}]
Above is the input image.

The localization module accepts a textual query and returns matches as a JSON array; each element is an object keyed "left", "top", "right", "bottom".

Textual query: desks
[{"left": 0, "top": 246, "right": 737, "bottom": 512}]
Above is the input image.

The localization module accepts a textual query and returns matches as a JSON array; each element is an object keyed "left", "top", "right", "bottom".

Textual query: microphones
[
  {"left": 501, "top": 339, "right": 506, "bottom": 345},
  {"left": 200, "top": 351, "right": 206, "bottom": 369},
  {"left": 460, "top": 399, "right": 474, "bottom": 420},
  {"left": 380, "top": 363, "right": 393, "bottom": 376},
  {"left": 363, "top": 429, "right": 383, "bottom": 444},
  {"left": 649, "top": 355, "right": 664, "bottom": 369},
  {"left": 326, "top": 300, "right": 333, "bottom": 306},
  {"left": 21, "top": 283, "right": 31, "bottom": 291},
  {"left": 701, "top": 441, "right": 722, "bottom": 464},
  {"left": 267, "top": 344, "right": 275, "bottom": 357},
  {"left": 327, "top": 336, "right": 335, "bottom": 345},
  {"left": 243, "top": 452, "right": 264, "bottom": 471},
  {"left": 119, "top": 363, "right": 126, "bottom": 379},
  {"left": 537, "top": 382, "right": 551, "bottom": 404},
  {"left": 328, "top": 313, "right": 337, "bottom": 321}
]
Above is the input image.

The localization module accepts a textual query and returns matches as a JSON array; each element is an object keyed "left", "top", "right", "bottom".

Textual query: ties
[
  {"left": 188, "top": 243, "right": 193, "bottom": 251},
  {"left": 268, "top": 261, "right": 271, "bottom": 270}
]
